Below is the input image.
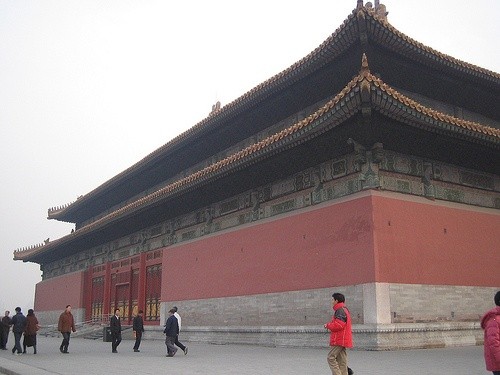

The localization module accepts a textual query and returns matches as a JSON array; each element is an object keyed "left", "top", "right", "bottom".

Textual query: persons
[
  {"left": 324, "top": 292, "right": 352, "bottom": 375},
  {"left": 481, "top": 290, "right": 500, "bottom": 375},
  {"left": 0, "top": 304, "right": 188, "bottom": 358}
]
